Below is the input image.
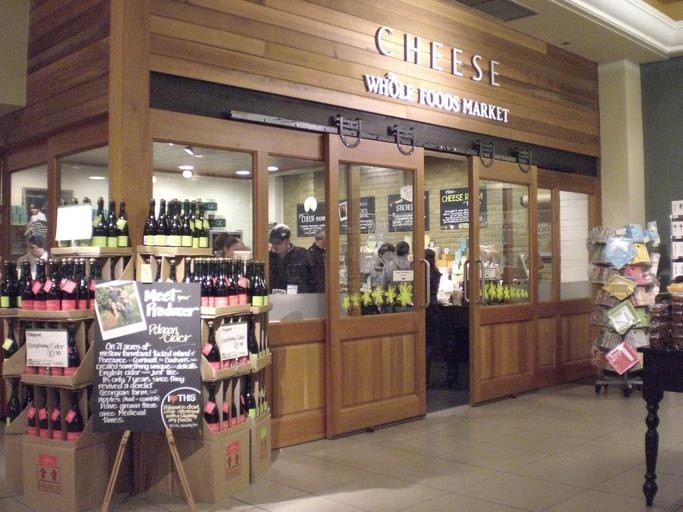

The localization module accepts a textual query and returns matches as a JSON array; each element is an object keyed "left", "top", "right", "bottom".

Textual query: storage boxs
[
  {"left": 133, "top": 431, "right": 250, "bottom": 504},
  {"left": 0, "top": 434, "right": 22, "bottom": 498},
  {"left": 21, "top": 441, "right": 130, "bottom": 512},
  {"left": 250, "top": 416, "right": 274, "bottom": 483}
]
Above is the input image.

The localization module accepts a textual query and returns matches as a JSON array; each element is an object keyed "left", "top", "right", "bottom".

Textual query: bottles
[
  {"left": 57, "top": 196, "right": 129, "bottom": 248},
  {"left": 141, "top": 198, "right": 210, "bottom": 249},
  {"left": 0, "top": 256, "right": 99, "bottom": 441},
  {"left": 144, "top": 256, "right": 269, "bottom": 433}
]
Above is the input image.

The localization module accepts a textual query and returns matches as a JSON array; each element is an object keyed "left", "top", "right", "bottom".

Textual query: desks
[
  {"left": 443, "top": 306, "right": 469, "bottom": 386},
  {"left": 637, "top": 345, "right": 683, "bottom": 506}
]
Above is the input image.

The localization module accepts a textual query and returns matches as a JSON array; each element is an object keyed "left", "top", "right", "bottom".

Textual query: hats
[{"left": 269, "top": 224, "right": 291, "bottom": 243}]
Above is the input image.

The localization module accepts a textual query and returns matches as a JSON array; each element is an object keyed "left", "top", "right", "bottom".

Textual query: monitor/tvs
[{"left": 208, "top": 230, "right": 243, "bottom": 252}]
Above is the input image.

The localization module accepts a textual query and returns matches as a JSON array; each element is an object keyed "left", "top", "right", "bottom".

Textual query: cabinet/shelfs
[
  {"left": 669, "top": 200, "right": 683, "bottom": 284},
  {"left": 588, "top": 221, "right": 661, "bottom": 398}
]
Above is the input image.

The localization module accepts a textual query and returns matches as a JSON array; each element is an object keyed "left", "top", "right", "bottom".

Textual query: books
[{"left": 585, "top": 220, "right": 661, "bottom": 375}]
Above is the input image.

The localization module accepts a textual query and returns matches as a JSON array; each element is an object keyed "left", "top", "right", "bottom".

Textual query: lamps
[
  {"left": 185, "top": 145, "right": 193, "bottom": 155},
  {"left": 183, "top": 170, "right": 193, "bottom": 179}
]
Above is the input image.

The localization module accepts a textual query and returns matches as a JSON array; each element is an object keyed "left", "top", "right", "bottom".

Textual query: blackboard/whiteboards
[
  {"left": 297, "top": 186, "right": 487, "bottom": 237},
  {"left": 90, "top": 280, "right": 204, "bottom": 434}
]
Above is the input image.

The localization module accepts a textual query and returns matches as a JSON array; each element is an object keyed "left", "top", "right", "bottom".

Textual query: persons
[
  {"left": 308, "top": 228, "right": 326, "bottom": 293},
  {"left": 16, "top": 234, "right": 48, "bottom": 283},
  {"left": 108, "top": 287, "right": 133, "bottom": 323},
  {"left": 368, "top": 240, "right": 441, "bottom": 344},
  {"left": 268, "top": 224, "right": 322, "bottom": 294},
  {"left": 25, "top": 204, "right": 48, "bottom": 229},
  {"left": 214, "top": 231, "right": 246, "bottom": 258}
]
